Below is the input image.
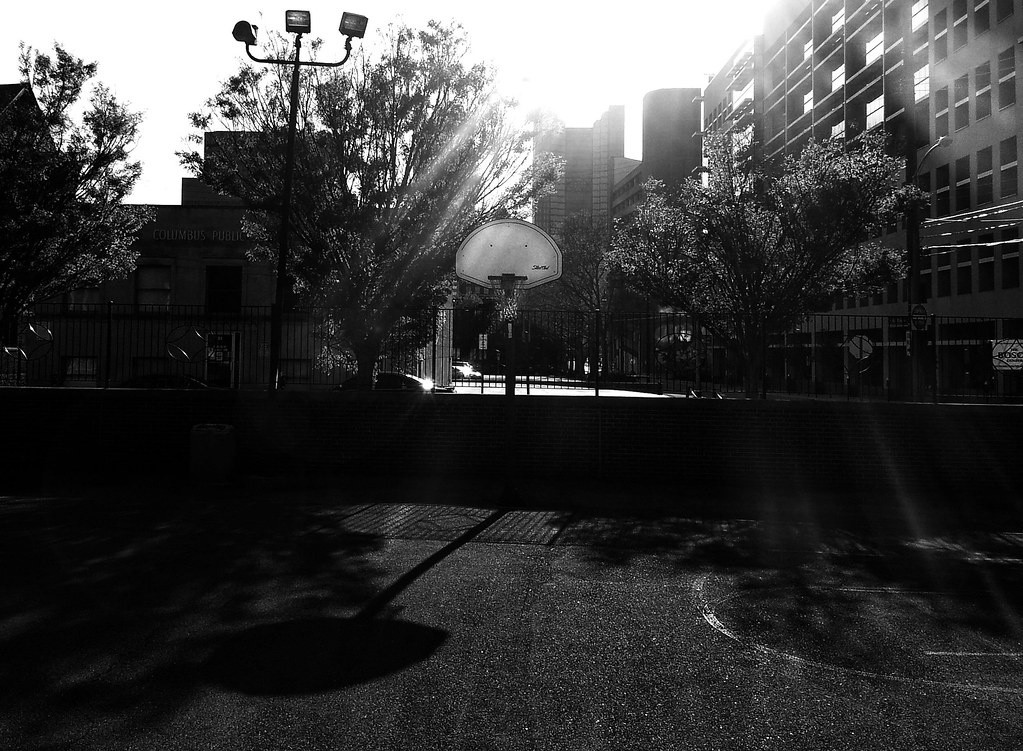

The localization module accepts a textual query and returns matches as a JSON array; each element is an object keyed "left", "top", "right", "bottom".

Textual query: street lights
[
  {"left": 229, "top": 8, "right": 370, "bottom": 389},
  {"left": 900, "top": 133, "right": 956, "bottom": 402}
]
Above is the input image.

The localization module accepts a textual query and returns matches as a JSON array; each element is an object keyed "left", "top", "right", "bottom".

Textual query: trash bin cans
[
  {"left": 190, "top": 423, "right": 234, "bottom": 488},
  {"left": 204, "top": 331, "right": 243, "bottom": 389}
]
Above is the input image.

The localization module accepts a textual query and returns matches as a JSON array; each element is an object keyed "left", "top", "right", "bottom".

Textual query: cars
[
  {"left": 454, "top": 365, "right": 482, "bottom": 380},
  {"left": 333, "top": 367, "right": 455, "bottom": 392},
  {"left": 105, "top": 374, "right": 209, "bottom": 389}
]
